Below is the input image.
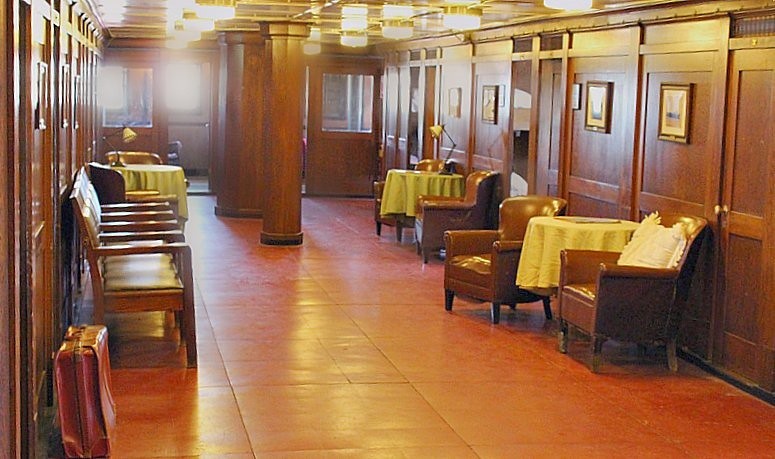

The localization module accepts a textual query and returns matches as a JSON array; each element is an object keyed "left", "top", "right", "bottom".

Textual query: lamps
[
  {"left": 429, "top": 123, "right": 457, "bottom": 175},
  {"left": 170, "top": 0, "right": 604, "bottom": 49},
  {"left": 102, "top": 124, "right": 137, "bottom": 167}
]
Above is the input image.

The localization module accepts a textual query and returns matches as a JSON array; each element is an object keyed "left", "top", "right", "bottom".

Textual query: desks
[
  {"left": 379, "top": 169, "right": 465, "bottom": 242},
  {"left": 102, "top": 163, "right": 188, "bottom": 233},
  {"left": 515, "top": 216, "right": 641, "bottom": 319}
]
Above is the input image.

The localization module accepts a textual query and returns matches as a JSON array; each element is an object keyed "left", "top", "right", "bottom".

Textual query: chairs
[
  {"left": 558, "top": 211, "right": 709, "bottom": 374},
  {"left": 373, "top": 159, "right": 446, "bottom": 241},
  {"left": 70, "top": 151, "right": 198, "bottom": 369},
  {"left": 416, "top": 169, "right": 499, "bottom": 264},
  {"left": 443, "top": 194, "right": 568, "bottom": 325}
]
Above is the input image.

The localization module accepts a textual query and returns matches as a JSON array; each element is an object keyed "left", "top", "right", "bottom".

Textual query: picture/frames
[
  {"left": 657, "top": 82, "right": 694, "bottom": 144},
  {"left": 62, "top": 64, "right": 70, "bottom": 128},
  {"left": 583, "top": 80, "right": 614, "bottom": 134},
  {"left": 481, "top": 85, "right": 499, "bottom": 124},
  {"left": 35, "top": 61, "right": 48, "bottom": 130},
  {"left": 448, "top": 87, "right": 461, "bottom": 118},
  {"left": 74, "top": 75, "right": 81, "bottom": 130}
]
